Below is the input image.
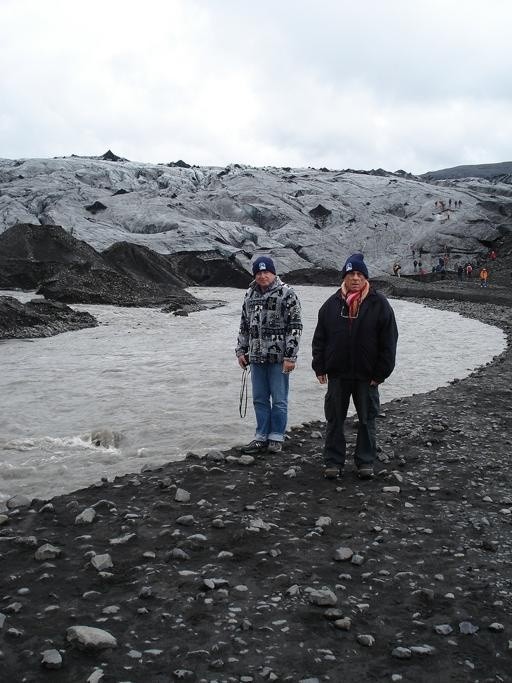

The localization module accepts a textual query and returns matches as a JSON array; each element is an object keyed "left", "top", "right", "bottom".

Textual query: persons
[
  {"left": 393, "top": 249, "right": 496, "bottom": 288},
  {"left": 447, "top": 210, "right": 450, "bottom": 219},
  {"left": 235, "top": 256, "right": 303, "bottom": 454},
  {"left": 434, "top": 198, "right": 463, "bottom": 209},
  {"left": 311, "top": 254, "right": 398, "bottom": 480}
]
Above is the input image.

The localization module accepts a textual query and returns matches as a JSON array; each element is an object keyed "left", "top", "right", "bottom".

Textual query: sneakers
[
  {"left": 241, "top": 440, "right": 266, "bottom": 455},
  {"left": 322, "top": 466, "right": 342, "bottom": 480},
  {"left": 357, "top": 466, "right": 376, "bottom": 481},
  {"left": 266, "top": 439, "right": 284, "bottom": 453}
]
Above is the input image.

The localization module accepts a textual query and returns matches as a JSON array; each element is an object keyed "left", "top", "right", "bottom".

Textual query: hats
[
  {"left": 340, "top": 253, "right": 369, "bottom": 280},
  {"left": 251, "top": 256, "right": 276, "bottom": 279}
]
